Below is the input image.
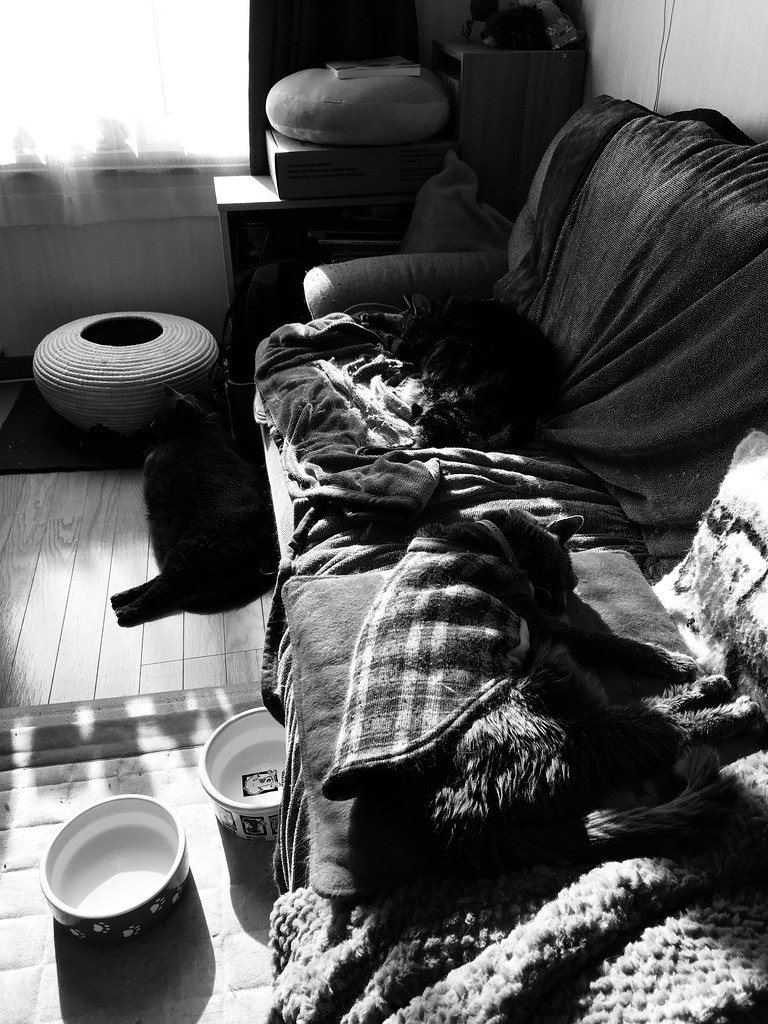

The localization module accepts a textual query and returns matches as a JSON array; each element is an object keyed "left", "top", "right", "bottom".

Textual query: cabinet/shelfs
[{"left": 210, "top": 39, "right": 588, "bottom": 324}]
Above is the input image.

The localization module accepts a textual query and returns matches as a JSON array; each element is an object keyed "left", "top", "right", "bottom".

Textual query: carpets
[
  {"left": 0, "top": 680, "right": 274, "bottom": 1023},
  {"left": 0, "top": 382, "right": 162, "bottom": 475}
]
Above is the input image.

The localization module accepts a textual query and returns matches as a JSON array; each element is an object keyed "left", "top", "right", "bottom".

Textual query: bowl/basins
[
  {"left": 38, "top": 794, "right": 187, "bottom": 943},
  {"left": 197, "top": 707, "right": 289, "bottom": 840}
]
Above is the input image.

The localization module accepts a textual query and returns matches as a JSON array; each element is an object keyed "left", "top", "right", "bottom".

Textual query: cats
[
  {"left": 111, "top": 386, "right": 274, "bottom": 623},
  {"left": 394, "top": 292, "right": 566, "bottom": 454},
  {"left": 358, "top": 509, "right": 763, "bottom": 874}
]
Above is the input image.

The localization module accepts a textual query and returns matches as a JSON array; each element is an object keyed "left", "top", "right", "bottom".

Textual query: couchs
[{"left": 243, "top": 91, "right": 768, "bottom": 1024}]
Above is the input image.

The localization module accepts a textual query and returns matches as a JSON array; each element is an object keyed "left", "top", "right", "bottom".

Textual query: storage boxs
[{"left": 262, "top": 127, "right": 458, "bottom": 201}]
